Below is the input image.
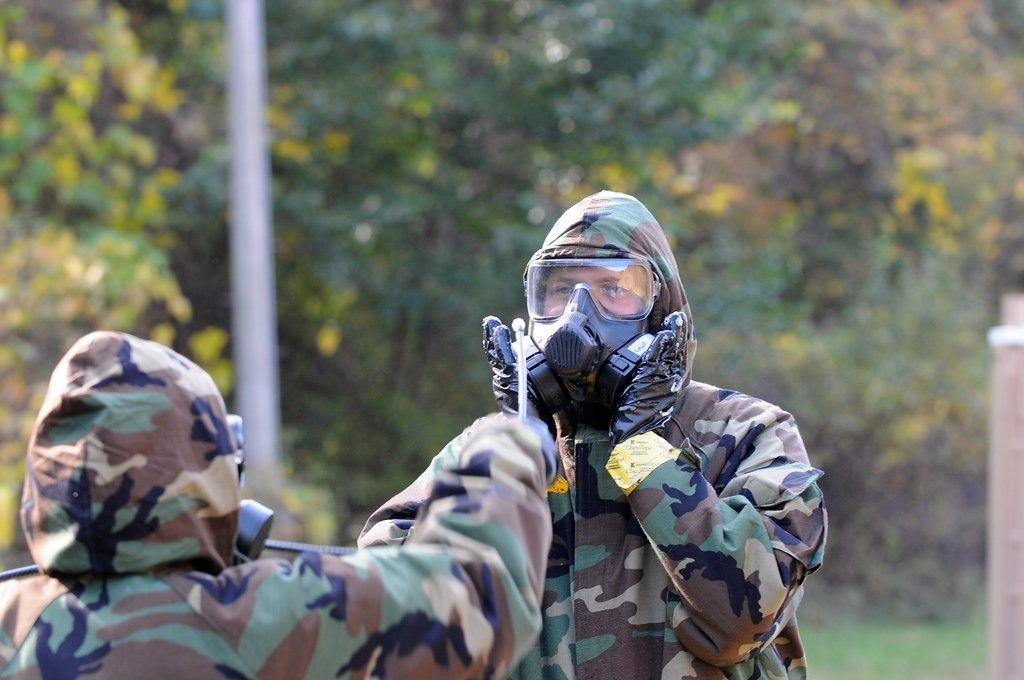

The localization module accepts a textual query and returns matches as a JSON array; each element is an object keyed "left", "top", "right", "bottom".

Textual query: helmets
[
  {"left": 16, "top": 328, "right": 273, "bottom": 567},
  {"left": 512, "top": 188, "right": 699, "bottom": 417}
]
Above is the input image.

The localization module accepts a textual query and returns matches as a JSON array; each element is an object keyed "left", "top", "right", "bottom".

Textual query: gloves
[
  {"left": 481, "top": 315, "right": 558, "bottom": 487},
  {"left": 608, "top": 311, "right": 688, "bottom": 449}
]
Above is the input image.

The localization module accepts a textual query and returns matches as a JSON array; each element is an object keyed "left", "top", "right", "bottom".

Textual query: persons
[
  {"left": 0, "top": 328, "right": 560, "bottom": 679},
  {"left": 357, "top": 190, "right": 827, "bottom": 680}
]
